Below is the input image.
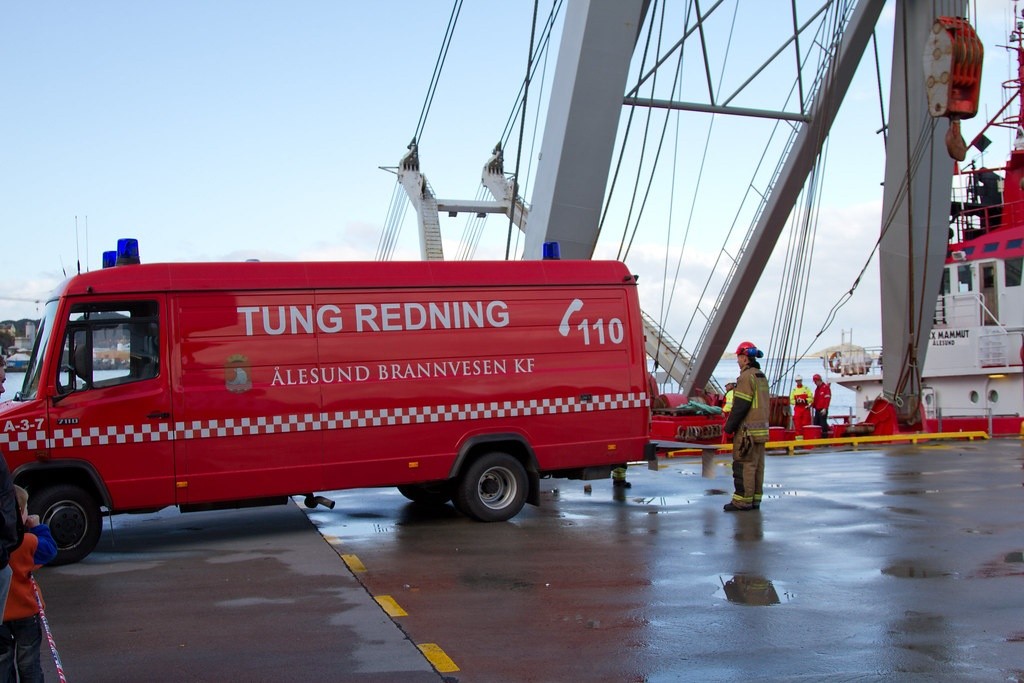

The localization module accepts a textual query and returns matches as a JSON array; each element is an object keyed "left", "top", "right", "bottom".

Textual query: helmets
[
  {"left": 813, "top": 374, "right": 821, "bottom": 381},
  {"left": 795, "top": 374, "right": 803, "bottom": 380},
  {"left": 735, "top": 342, "right": 756, "bottom": 355}
]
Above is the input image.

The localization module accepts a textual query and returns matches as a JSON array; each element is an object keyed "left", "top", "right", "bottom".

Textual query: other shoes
[{"left": 724, "top": 501, "right": 760, "bottom": 510}]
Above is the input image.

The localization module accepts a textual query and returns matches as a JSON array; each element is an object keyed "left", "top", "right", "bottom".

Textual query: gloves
[{"left": 797, "top": 399, "right": 826, "bottom": 416}]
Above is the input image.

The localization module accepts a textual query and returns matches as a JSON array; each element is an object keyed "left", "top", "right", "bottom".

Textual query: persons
[
  {"left": 789, "top": 375, "right": 813, "bottom": 435},
  {"left": 0, "top": 353, "right": 24, "bottom": 626},
  {"left": 613, "top": 463, "right": 632, "bottom": 487},
  {"left": 805, "top": 374, "right": 831, "bottom": 438},
  {"left": 721, "top": 383, "right": 737, "bottom": 444},
  {"left": 1, "top": 484, "right": 58, "bottom": 683},
  {"left": 722, "top": 342, "right": 770, "bottom": 510}
]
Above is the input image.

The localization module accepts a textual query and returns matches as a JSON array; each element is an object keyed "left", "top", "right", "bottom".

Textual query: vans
[{"left": 0, "top": 238, "right": 653, "bottom": 566}]
